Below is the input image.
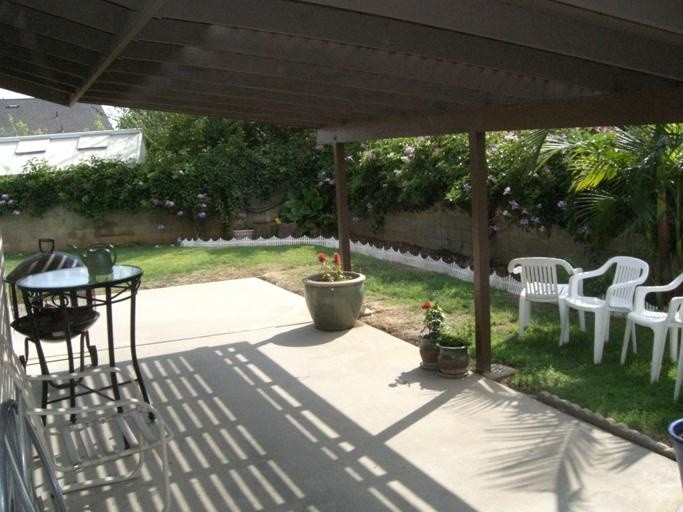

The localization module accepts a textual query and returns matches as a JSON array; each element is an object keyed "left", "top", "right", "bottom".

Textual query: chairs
[{"left": 506, "top": 254, "right": 682, "bottom": 403}]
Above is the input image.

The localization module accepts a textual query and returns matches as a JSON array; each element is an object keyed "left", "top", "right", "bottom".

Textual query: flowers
[
  {"left": 314, "top": 250, "right": 344, "bottom": 281},
  {"left": 421, "top": 302, "right": 441, "bottom": 339}
]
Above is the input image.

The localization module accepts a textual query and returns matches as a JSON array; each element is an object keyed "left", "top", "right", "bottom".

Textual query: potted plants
[{"left": 436, "top": 335, "right": 473, "bottom": 379}]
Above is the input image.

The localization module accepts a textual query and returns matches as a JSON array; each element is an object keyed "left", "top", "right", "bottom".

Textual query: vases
[{"left": 419, "top": 335, "right": 438, "bottom": 371}]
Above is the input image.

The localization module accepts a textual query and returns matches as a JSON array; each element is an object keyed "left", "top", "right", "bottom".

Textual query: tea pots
[{"left": 67, "top": 240, "right": 118, "bottom": 275}]
